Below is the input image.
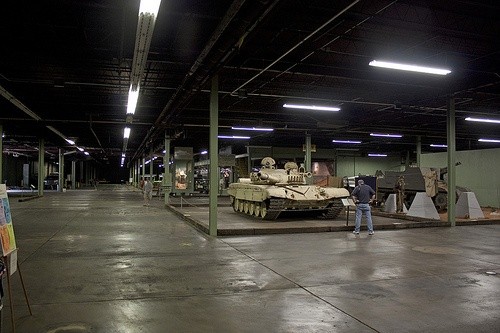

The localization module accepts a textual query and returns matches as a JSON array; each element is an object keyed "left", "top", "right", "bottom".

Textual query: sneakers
[
  {"left": 369, "top": 231, "right": 375, "bottom": 234},
  {"left": 352, "top": 231, "right": 359, "bottom": 234}
]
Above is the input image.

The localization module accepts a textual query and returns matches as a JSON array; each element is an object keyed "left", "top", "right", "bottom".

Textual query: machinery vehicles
[
  {"left": 376, "top": 161, "right": 471, "bottom": 210},
  {"left": 227, "top": 157, "right": 350, "bottom": 220}
]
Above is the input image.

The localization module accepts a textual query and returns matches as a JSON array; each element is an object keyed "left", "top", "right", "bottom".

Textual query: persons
[
  {"left": 142, "top": 178, "right": 154, "bottom": 207},
  {"left": 350, "top": 180, "right": 376, "bottom": 235}
]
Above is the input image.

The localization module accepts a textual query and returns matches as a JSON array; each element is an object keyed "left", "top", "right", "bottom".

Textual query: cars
[{"left": 43, "top": 172, "right": 59, "bottom": 190}]
